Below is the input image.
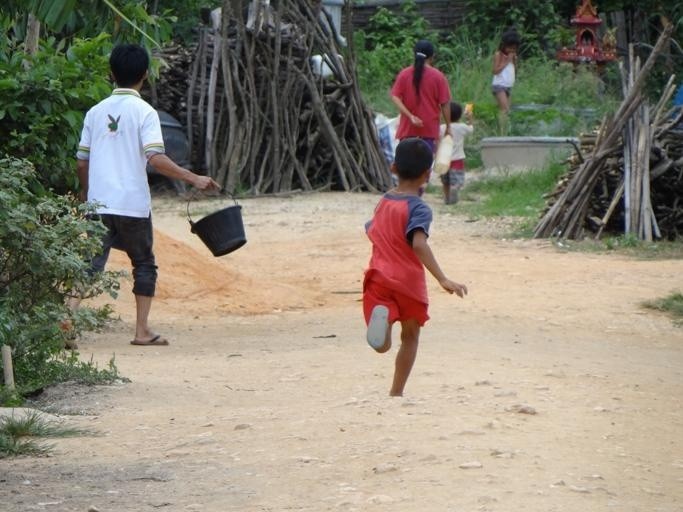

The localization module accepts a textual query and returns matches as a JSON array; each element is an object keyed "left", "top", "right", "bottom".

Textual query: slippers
[{"left": 131, "top": 335, "right": 169, "bottom": 345}]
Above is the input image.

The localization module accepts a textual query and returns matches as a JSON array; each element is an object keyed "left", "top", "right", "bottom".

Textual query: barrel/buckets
[
  {"left": 185, "top": 187, "right": 249, "bottom": 259},
  {"left": 432, "top": 134, "right": 455, "bottom": 176}
]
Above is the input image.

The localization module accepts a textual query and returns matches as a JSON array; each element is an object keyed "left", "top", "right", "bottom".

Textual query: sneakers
[
  {"left": 367, "top": 304, "right": 389, "bottom": 349},
  {"left": 444, "top": 188, "right": 458, "bottom": 204}
]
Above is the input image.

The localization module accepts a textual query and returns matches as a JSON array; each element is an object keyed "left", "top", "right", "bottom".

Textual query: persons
[
  {"left": 68, "top": 44, "right": 223, "bottom": 346},
  {"left": 440, "top": 102, "right": 474, "bottom": 205},
  {"left": 491, "top": 30, "right": 520, "bottom": 121},
  {"left": 362, "top": 138, "right": 468, "bottom": 397},
  {"left": 391, "top": 40, "right": 454, "bottom": 197}
]
